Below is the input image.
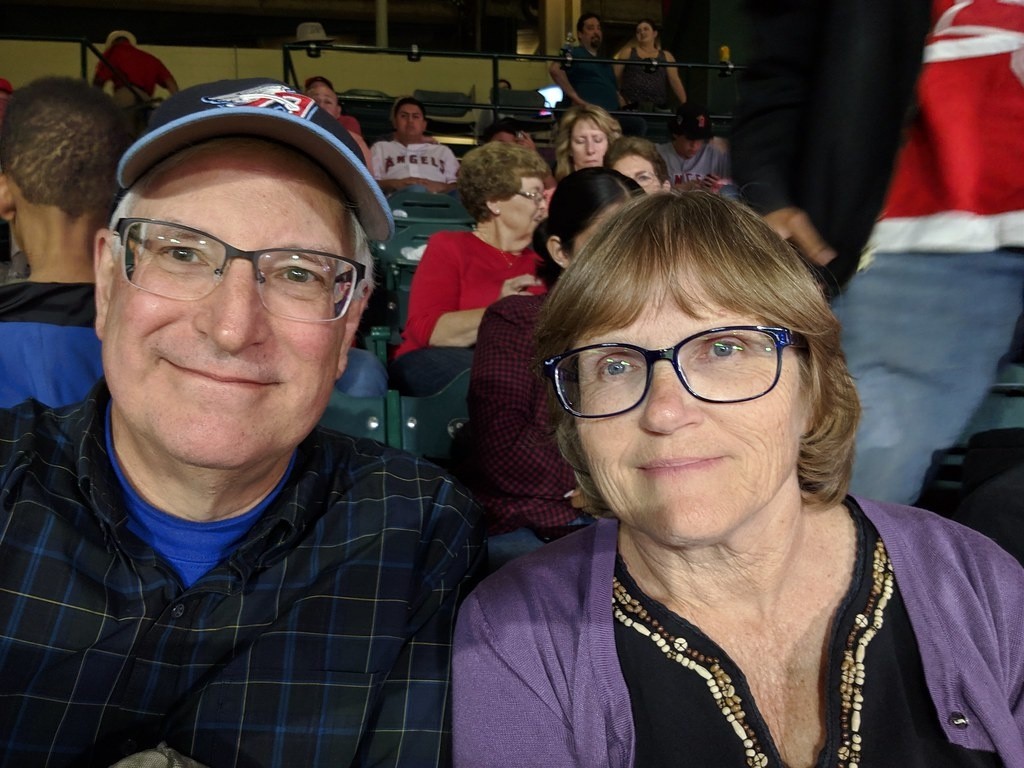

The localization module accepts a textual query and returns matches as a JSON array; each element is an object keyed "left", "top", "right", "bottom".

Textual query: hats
[
  {"left": 115, "top": 77, "right": 398, "bottom": 241},
  {"left": 675, "top": 102, "right": 714, "bottom": 141}
]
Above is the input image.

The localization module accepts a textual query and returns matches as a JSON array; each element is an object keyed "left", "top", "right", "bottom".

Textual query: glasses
[
  {"left": 540, "top": 323, "right": 807, "bottom": 419},
  {"left": 516, "top": 188, "right": 547, "bottom": 204},
  {"left": 113, "top": 217, "right": 367, "bottom": 324}
]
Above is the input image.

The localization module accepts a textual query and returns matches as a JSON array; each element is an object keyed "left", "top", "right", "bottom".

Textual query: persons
[
  {"left": 302, "top": 17, "right": 729, "bottom": 396},
  {"left": 91, "top": 31, "right": 180, "bottom": 108},
  {"left": 0, "top": 78, "right": 131, "bottom": 410},
  {"left": 468, "top": 166, "right": 648, "bottom": 575},
  {"left": 730, "top": 0, "right": 1024, "bottom": 510},
  {"left": 0, "top": 78, "right": 491, "bottom": 768},
  {"left": 453, "top": 181, "right": 1024, "bottom": 768}
]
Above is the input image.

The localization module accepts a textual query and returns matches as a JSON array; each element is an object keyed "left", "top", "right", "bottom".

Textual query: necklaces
[
  {"left": 476, "top": 229, "right": 520, "bottom": 269},
  {"left": 641, "top": 46, "right": 656, "bottom": 53}
]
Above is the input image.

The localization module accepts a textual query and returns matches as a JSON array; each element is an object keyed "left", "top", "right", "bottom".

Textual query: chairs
[
  {"left": 314, "top": 185, "right": 475, "bottom": 467},
  {"left": 335, "top": 82, "right": 648, "bottom": 149},
  {"left": 930, "top": 363, "right": 1024, "bottom": 504}
]
